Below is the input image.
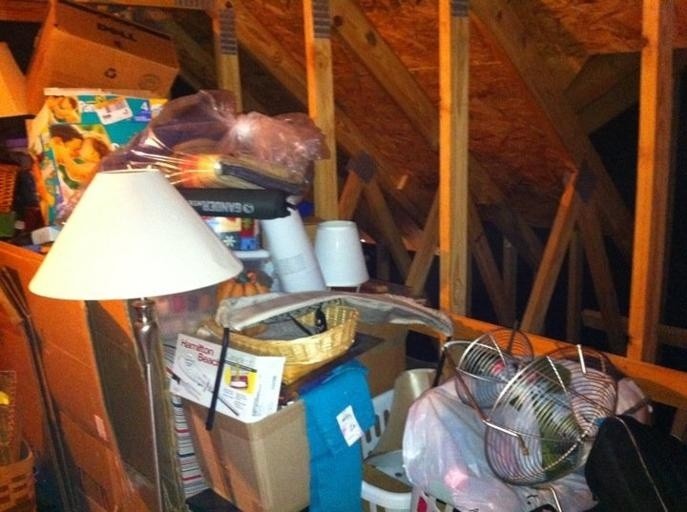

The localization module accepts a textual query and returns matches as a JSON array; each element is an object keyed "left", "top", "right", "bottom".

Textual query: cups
[{"left": 313, "top": 221, "right": 372, "bottom": 287}]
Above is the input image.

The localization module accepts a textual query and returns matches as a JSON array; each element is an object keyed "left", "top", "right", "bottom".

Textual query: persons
[
  {"left": 56, "top": 135, "right": 111, "bottom": 226},
  {"left": 49, "top": 124, "right": 83, "bottom": 165}
]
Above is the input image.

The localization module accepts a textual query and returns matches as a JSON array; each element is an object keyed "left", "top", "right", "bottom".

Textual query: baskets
[{"left": 204, "top": 291, "right": 360, "bottom": 380}]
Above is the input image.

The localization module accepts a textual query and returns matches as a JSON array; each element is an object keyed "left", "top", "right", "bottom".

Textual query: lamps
[{"left": 27, "top": 171, "right": 244, "bottom": 511}]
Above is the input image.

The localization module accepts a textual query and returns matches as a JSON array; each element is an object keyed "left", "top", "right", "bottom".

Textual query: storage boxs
[
  {"left": 25, "top": 0, "right": 180, "bottom": 117},
  {"left": 179, "top": 322, "right": 408, "bottom": 512},
  {"left": 168, "top": 333, "right": 286, "bottom": 424}
]
